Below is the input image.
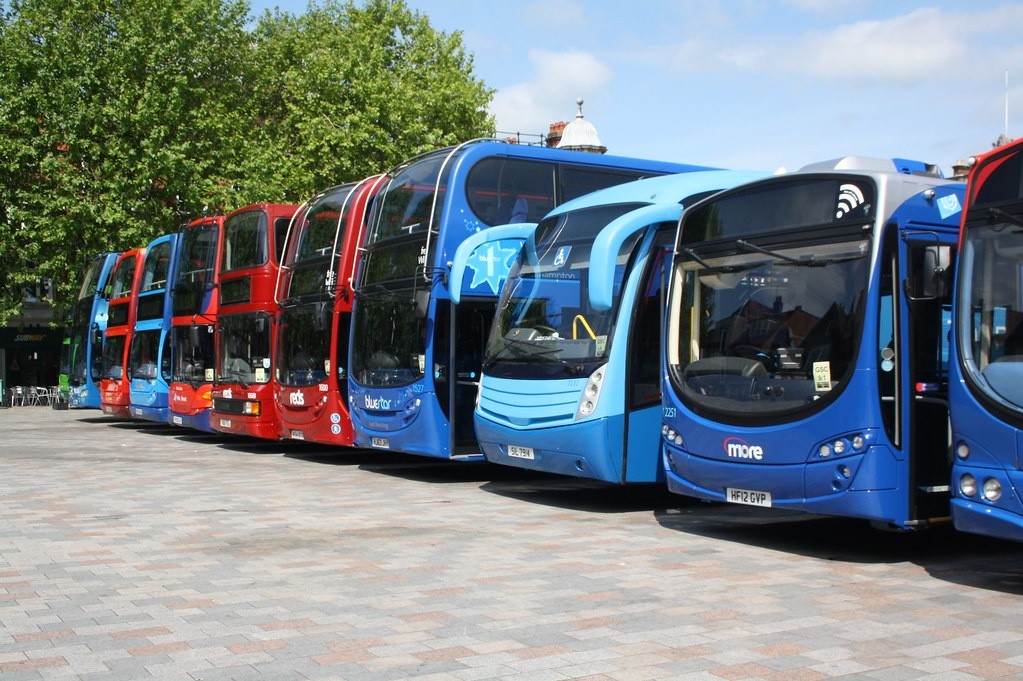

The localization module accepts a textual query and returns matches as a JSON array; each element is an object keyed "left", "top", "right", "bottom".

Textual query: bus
[
  {"left": 59, "top": 138, "right": 736, "bottom": 462},
  {"left": 449, "top": 168, "right": 797, "bottom": 484},
  {"left": 660, "top": 154, "right": 1006, "bottom": 528},
  {"left": 947, "top": 138, "right": 1023, "bottom": 542}
]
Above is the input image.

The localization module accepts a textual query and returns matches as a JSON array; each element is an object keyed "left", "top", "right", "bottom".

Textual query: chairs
[{"left": 8, "top": 385, "right": 72, "bottom": 409}]
